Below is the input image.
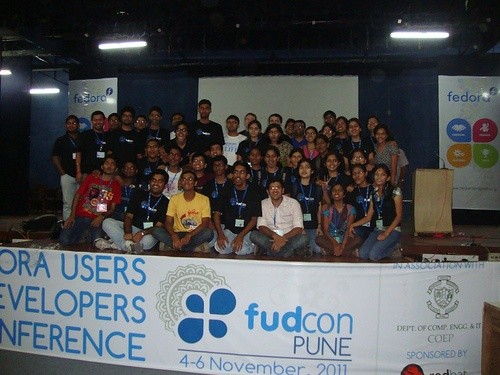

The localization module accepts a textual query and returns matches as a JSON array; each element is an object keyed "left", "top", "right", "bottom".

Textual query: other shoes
[
  {"left": 320, "top": 248, "right": 330, "bottom": 257},
  {"left": 92, "top": 238, "right": 118, "bottom": 250},
  {"left": 299, "top": 248, "right": 313, "bottom": 259},
  {"left": 392, "top": 243, "right": 404, "bottom": 258},
  {"left": 349, "top": 248, "right": 359, "bottom": 258},
  {"left": 193, "top": 241, "right": 211, "bottom": 254},
  {"left": 254, "top": 245, "right": 266, "bottom": 257},
  {"left": 158, "top": 241, "right": 174, "bottom": 252},
  {"left": 127, "top": 242, "right": 145, "bottom": 254}
]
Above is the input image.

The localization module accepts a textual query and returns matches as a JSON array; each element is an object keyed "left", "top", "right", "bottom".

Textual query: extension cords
[{"left": 422, "top": 253, "right": 479, "bottom": 263}]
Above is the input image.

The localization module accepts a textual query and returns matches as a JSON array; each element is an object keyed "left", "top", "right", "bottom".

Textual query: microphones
[{"left": 434, "top": 153, "right": 445, "bottom": 168}]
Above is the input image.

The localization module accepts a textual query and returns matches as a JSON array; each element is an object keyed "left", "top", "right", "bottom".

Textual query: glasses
[
  {"left": 67, "top": 122, "right": 77, "bottom": 125},
  {"left": 181, "top": 177, "right": 193, "bottom": 182},
  {"left": 122, "top": 115, "right": 131, "bottom": 118},
  {"left": 135, "top": 120, "right": 145, "bottom": 124},
  {"left": 150, "top": 114, "right": 160, "bottom": 118},
  {"left": 323, "top": 130, "right": 333, "bottom": 134},
  {"left": 269, "top": 186, "right": 281, "bottom": 190},
  {"left": 176, "top": 128, "right": 187, "bottom": 132},
  {"left": 351, "top": 171, "right": 362, "bottom": 176},
  {"left": 294, "top": 125, "right": 304, "bottom": 129},
  {"left": 352, "top": 156, "right": 363, "bottom": 161},
  {"left": 233, "top": 170, "right": 246, "bottom": 175},
  {"left": 193, "top": 159, "right": 204, "bottom": 164},
  {"left": 110, "top": 119, "right": 118, "bottom": 122}
]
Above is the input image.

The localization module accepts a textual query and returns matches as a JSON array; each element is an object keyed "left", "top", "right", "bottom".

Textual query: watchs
[{"left": 141, "top": 231, "right": 145, "bottom": 236}]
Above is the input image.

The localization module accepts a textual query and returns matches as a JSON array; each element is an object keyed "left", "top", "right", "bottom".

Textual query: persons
[
  {"left": 246, "top": 178, "right": 310, "bottom": 258},
  {"left": 153, "top": 170, "right": 213, "bottom": 254},
  {"left": 313, "top": 183, "right": 365, "bottom": 259},
  {"left": 51, "top": 95, "right": 409, "bottom": 257},
  {"left": 91, "top": 169, "right": 170, "bottom": 256},
  {"left": 210, "top": 162, "right": 264, "bottom": 256},
  {"left": 57, "top": 154, "right": 124, "bottom": 247},
  {"left": 348, "top": 165, "right": 405, "bottom": 262}
]
